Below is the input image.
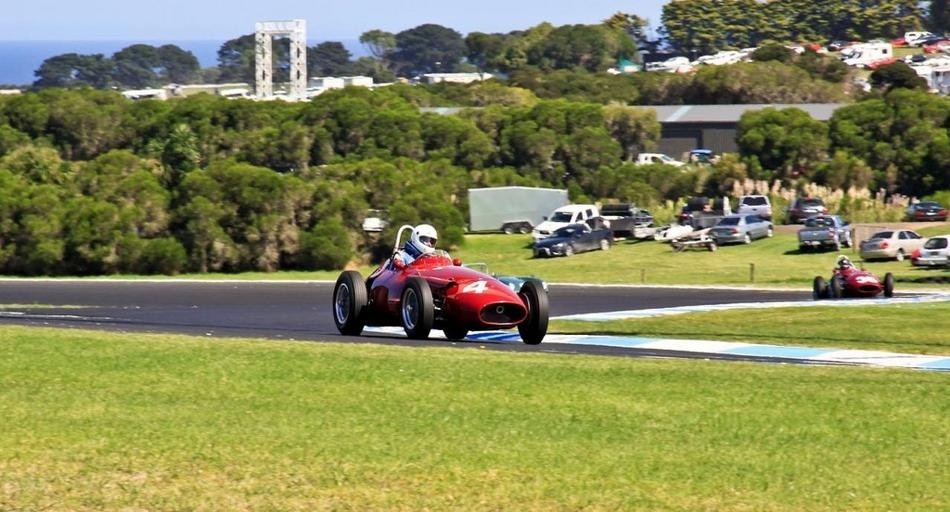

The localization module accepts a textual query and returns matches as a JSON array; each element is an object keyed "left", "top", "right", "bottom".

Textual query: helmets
[
  {"left": 838, "top": 258, "right": 850, "bottom": 267},
  {"left": 411, "top": 223, "right": 437, "bottom": 254}
]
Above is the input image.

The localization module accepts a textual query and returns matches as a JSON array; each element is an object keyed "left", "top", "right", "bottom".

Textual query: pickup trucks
[{"left": 797, "top": 215, "right": 855, "bottom": 252}]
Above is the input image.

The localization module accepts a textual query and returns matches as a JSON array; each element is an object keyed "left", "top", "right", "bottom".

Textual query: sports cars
[
  {"left": 814, "top": 264, "right": 897, "bottom": 299},
  {"left": 332, "top": 223, "right": 550, "bottom": 347}
]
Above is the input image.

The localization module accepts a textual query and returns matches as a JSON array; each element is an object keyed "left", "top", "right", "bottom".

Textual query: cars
[
  {"left": 911, "top": 234, "right": 950, "bottom": 269},
  {"left": 638, "top": 153, "right": 686, "bottom": 168},
  {"left": 623, "top": 46, "right": 759, "bottom": 76},
  {"left": 829, "top": 30, "right": 949, "bottom": 95},
  {"left": 907, "top": 202, "right": 947, "bottom": 221},
  {"left": 712, "top": 215, "right": 773, "bottom": 246},
  {"left": 534, "top": 221, "right": 614, "bottom": 257},
  {"left": 606, "top": 201, "right": 729, "bottom": 242},
  {"left": 861, "top": 230, "right": 927, "bottom": 261}
]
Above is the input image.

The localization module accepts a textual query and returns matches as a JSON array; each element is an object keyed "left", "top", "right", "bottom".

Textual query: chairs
[
  {"left": 391, "top": 224, "right": 419, "bottom": 272},
  {"left": 835, "top": 255, "right": 856, "bottom": 270}
]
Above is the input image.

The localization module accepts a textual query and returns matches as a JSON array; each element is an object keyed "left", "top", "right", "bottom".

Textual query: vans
[
  {"left": 738, "top": 194, "right": 773, "bottom": 222},
  {"left": 688, "top": 149, "right": 715, "bottom": 165}
]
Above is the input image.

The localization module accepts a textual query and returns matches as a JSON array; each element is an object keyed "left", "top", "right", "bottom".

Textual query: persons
[
  {"left": 392, "top": 224, "right": 447, "bottom": 268},
  {"left": 836, "top": 255, "right": 854, "bottom": 275}
]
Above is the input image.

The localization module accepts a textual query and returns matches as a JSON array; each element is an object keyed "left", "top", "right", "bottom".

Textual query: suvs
[
  {"left": 791, "top": 197, "right": 826, "bottom": 222},
  {"left": 532, "top": 204, "right": 600, "bottom": 244}
]
